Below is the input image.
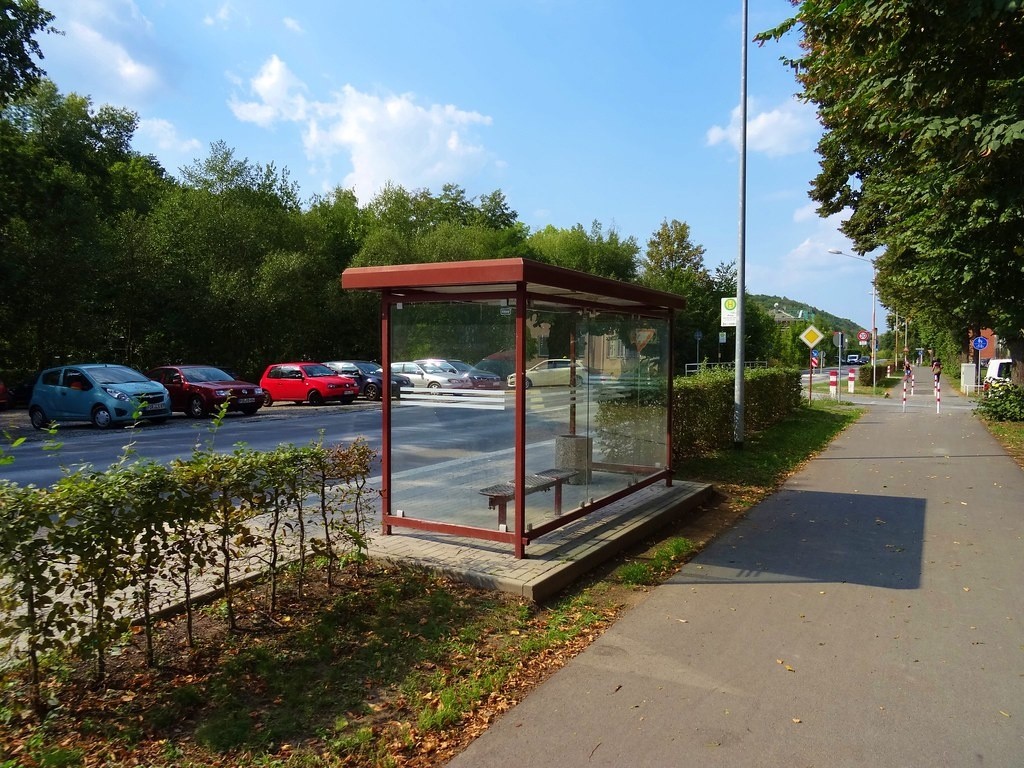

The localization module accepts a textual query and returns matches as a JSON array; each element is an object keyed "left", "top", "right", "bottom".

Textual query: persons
[{"left": 903, "top": 356, "right": 941, "bottom": 382}]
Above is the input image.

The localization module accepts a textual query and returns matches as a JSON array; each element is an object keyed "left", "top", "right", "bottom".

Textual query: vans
[{"left": 984, "top": 358, "right": 1012, "bottom": 398}]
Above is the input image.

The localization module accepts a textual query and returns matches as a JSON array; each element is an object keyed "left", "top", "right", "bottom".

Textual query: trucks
[{"left": 847, "top": 354, "right": 859, "bottom": 364}]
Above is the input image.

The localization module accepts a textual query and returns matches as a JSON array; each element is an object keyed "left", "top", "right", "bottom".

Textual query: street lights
[
  {"left": 888, "top": 312, "right": 908, "bottom": 364},
  {"left": 827, "top": 248, "right": 877, "bottom": 386},
  {"left": 868, "top": 291, "right": 899, "bottom": 371}
]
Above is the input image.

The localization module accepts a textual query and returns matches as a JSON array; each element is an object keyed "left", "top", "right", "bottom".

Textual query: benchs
[{"left": 478, "top": 469, "right": 581, "bottom": 531}]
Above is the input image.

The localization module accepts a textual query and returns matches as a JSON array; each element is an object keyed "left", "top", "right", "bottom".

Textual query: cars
[
  {"left": 258, "top": 362, "right": 360, "bottom": 406},
  {"left": 140, "top": 363, "right": 268, "bottom": 419},
  {"left": 506, "top": 357, "right": 587, "bottom": 389},
  {"left": 858, "top": 356, "right": 870, "bottom": 364},
  {"left": 371, "top": 360, "right": 472, "bottom": 398},
  {"left": 413, "top": 359, "right": 502, "bottom": 392},
  {"left": 312, "top": 358, "right": 414, "bottom": 402},
  {"left": 29, "top": 361, "right": 172, "bottom": 431}
]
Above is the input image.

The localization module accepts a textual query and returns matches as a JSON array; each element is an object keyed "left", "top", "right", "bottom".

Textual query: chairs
[{"left": 67, "top": 375, "right": 83, "bottom": 388}]
